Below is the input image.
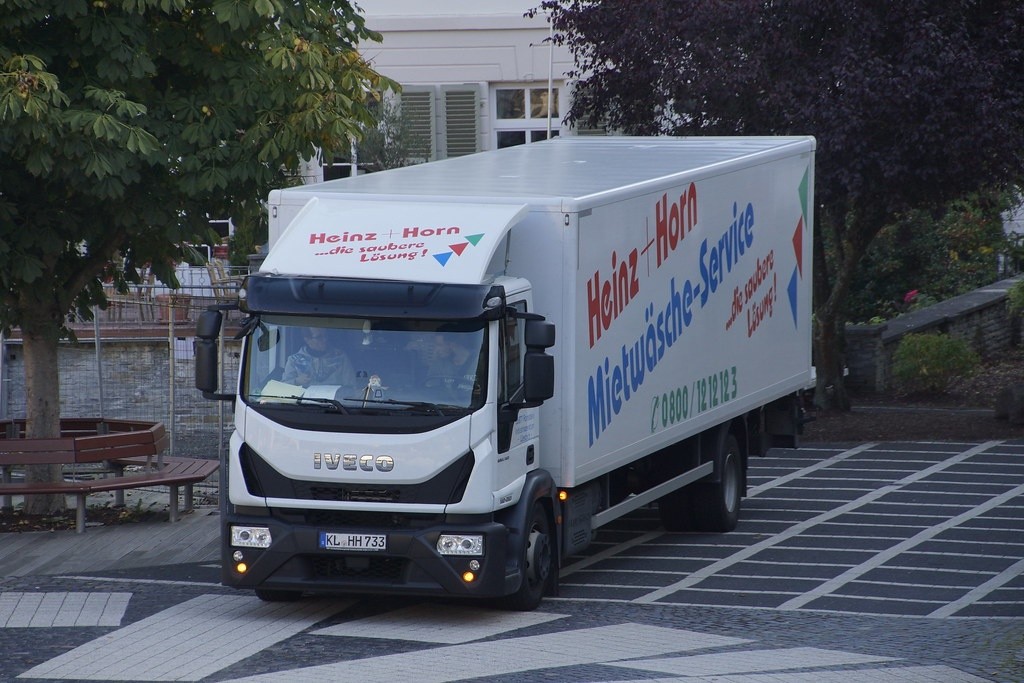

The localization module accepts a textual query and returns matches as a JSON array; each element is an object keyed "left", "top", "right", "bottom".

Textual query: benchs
[{"left": 0, "top": 412, "right": 222, "bottom": 525}]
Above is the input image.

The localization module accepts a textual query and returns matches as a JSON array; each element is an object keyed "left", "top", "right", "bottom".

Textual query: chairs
[
  {"left": 104, "top": 262, "right": 155, "bottom": 322},
  {"left": 214, "top": 259, "right": 239, "bottom": 320},
  {"left": 206, "top": 263, "right": 246, "bottom": 321}
]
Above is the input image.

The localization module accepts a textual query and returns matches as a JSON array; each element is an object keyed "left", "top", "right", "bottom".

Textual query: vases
[{"left": 157, "top": 293, "right": 191, "bottom": 322}]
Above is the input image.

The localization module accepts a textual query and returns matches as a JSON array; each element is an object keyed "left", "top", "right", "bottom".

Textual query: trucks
[{"left": 193, "top": 133, "right": 821, "bottom": 611}]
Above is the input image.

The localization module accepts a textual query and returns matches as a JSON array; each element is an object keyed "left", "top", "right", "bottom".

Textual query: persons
[
  {"left": 424, "top": 332, "right": 486, "bottom": 400},
  {"left": 280, "top": 325, "right": 357, "bottom": 388}
]
